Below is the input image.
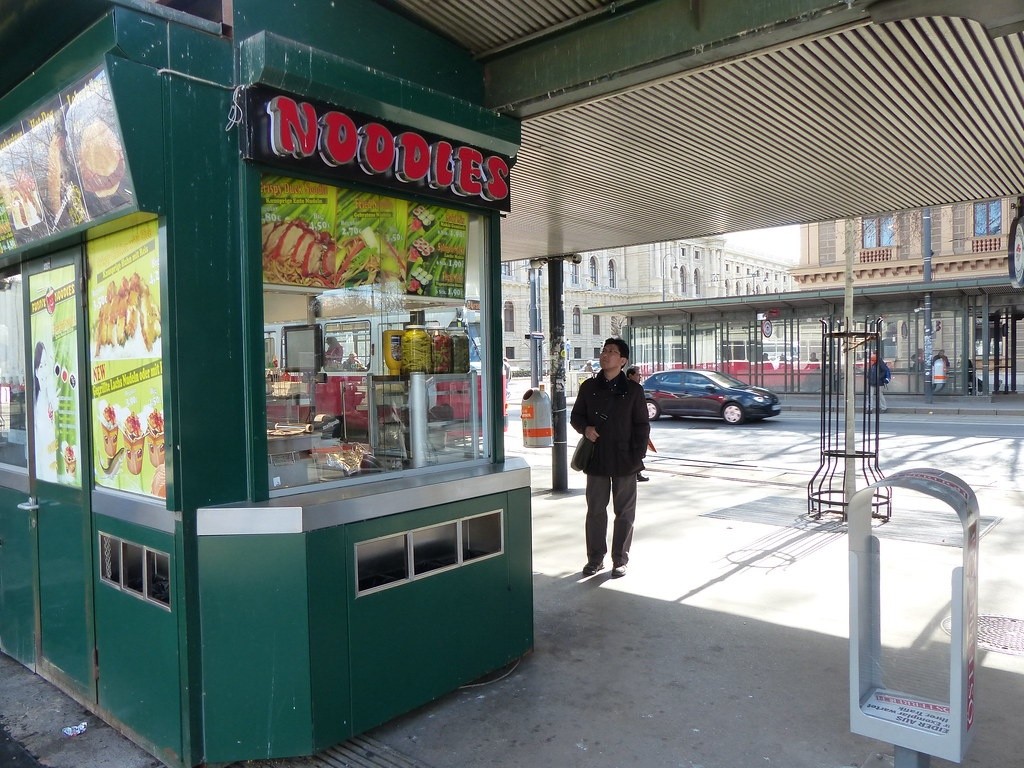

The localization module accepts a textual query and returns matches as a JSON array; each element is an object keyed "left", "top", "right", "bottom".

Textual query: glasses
[{"left": 600, "top": 348, "right": 621, "bottom": 356}]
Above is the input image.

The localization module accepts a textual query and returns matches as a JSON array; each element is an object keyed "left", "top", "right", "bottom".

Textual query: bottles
[
  {"left": 382, "top": 330, "right": 405, "bottom": 376},
  {"left": 400, "top": 325, "right": 432, "bottom": 376},
  {"left": 426, "top": 326, "right": 453, "bottom": 373},
  {"left": 446, "top": 327, "right": 470, "bottom": 373}
]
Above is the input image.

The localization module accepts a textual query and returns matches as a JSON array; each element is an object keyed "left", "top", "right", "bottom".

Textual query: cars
[
  {"left": 580, "top": 361, "right": 600, "bottom": 371},
  {"left": 641, "top": 370, "right": 782, "bottom": 424}
]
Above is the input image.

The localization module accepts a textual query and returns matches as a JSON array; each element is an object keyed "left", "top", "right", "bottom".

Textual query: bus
[{"left": 263, "top": 312, "right": 509, "bottom": 451}]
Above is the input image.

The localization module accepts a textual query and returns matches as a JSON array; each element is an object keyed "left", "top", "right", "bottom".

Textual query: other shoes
[
  {"left": 637, "top": 473, "right": 649, "bottom": 481},
  {"left": 582, "top": 561, "right": 605, "bottom": 575},
  {"left": 612, "top": 563, "right": 627, "bottom": 576}
]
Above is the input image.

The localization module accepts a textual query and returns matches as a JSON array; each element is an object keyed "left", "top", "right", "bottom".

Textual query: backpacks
[{"left": 882, "top": 362, "right": 892, "bottom": 391}]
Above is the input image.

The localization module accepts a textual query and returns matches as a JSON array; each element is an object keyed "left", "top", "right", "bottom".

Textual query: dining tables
[{"left": 267, "top": 427, "right": 322, "bottom": 490}]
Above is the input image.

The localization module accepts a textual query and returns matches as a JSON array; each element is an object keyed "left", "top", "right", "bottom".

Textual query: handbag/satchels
[{"left": 570, "top": 435, "right": 594, "bottom": 471}]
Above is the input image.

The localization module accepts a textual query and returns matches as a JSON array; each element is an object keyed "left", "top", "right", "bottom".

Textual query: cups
[
  {"left": 99, "top": 417, "right": 119, "bottom": 458},
  {"left": 120, "top": 426, "right": 147, "bottom": 475},
  {"left": 145, "top": 427, "right": 165, "bottom": 469}
]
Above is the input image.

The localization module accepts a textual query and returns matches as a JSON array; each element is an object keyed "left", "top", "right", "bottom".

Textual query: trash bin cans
[
  {"left": 933, "top": 357, "right": 947, "bottom": 384},
  {"left": 520, "top": 385, "right": 554, "bottom": 448}
]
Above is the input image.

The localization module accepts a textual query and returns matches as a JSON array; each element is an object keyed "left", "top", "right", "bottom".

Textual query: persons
[
  {"left": 960, "top": 354, "right": 974, "bottom": 394},
  {"left": 503, "top": 358, "right": 512, "bottom": 397},
  {"left": 932, "top": 350, "right": 950, "bottom": 393},
  {"left": 343, "top": 353, "right": 364, "bottom": 369},
  {"left": 866, "top": 350, "right": 887, "bottom": 414},
  {"left": 34, "top": 342, "right": 61, "bottom": 481},
  {"left": 584, "top": 360, "right": 593, "bottom": 372},
  {"left": 626, "top": 366, "right": 650, "bottom": 481},
  {"left": 570, "top": 338, "right": 651, "bottom": 576},
  {"left": 804, "top": 353, "right": 820, "bottom": 369},
  {"left": 326, "top": 337, "right": 343, "bottom": 366},
  {"left": 911, "top": 354, "right": 925, "bottom": 371}
]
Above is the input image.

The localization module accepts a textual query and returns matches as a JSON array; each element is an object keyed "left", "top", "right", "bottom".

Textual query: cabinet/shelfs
[{"left": 366, "top": 369, "right": 479, "bottom": 470}]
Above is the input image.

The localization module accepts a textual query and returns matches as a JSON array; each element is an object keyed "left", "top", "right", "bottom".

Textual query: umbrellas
[{"left": 647, "top": 438, "right": 657, "bottom": 453}]
